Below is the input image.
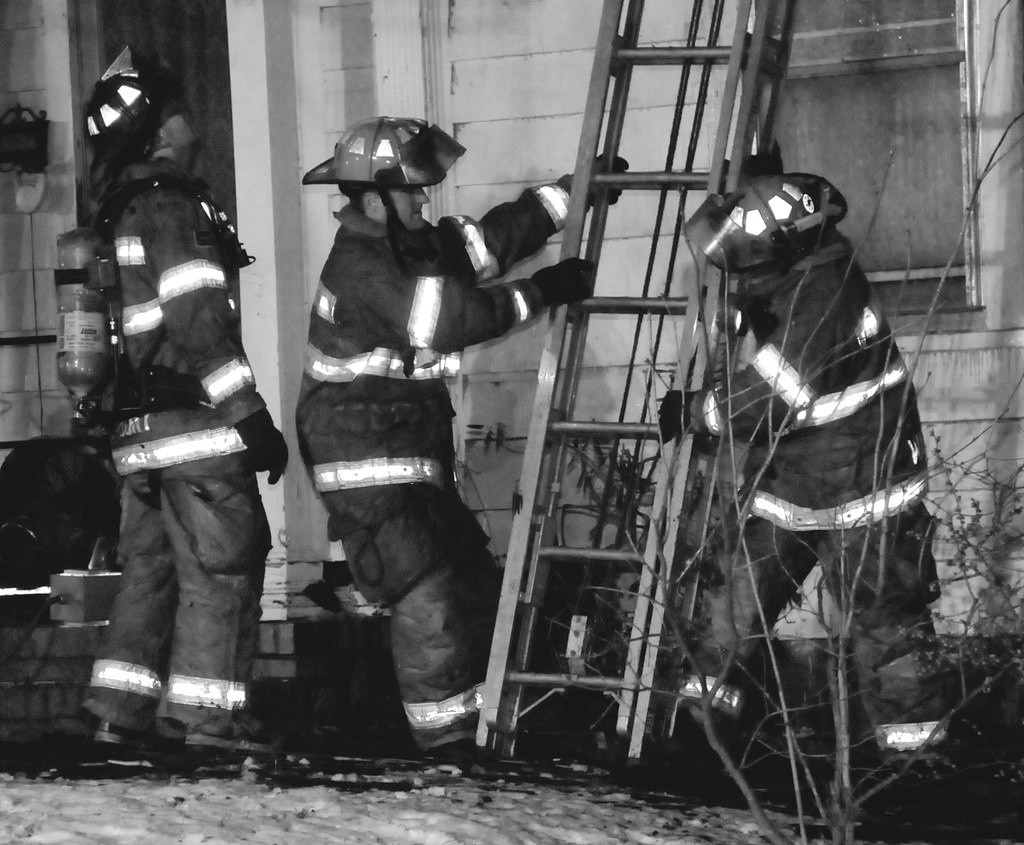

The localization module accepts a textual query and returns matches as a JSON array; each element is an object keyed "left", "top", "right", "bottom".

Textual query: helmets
[
  {"left": 302, "top": 116, "right": 467, "bottom": 185},
  {"left": 684, "top": 172, "right": 850, "bottom": 273},
  {"left": 84, "top": 34, "right": 193, "bottom": 166}
]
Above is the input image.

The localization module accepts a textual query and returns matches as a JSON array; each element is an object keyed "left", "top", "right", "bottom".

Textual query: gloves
[
  {"left": 658, "top": 390, "right": 697, "bottom": 431},
  {"left": 233, "top": 407, "right": 289, "bottom": 485},
  {"left": 529, "top": 258, "right": 596, "bottom": 306},
  {"left": 571, "top": 154, "right": 629, "bottom": 206}
]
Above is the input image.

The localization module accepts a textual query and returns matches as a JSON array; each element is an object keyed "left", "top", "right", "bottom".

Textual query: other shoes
[
  {"left": 424, "top": 738, "right": 476, "bottom": 774},
  {"left": 92, "top": 721, "right": 187, "bottom": 760},
  {"left": 186, "top": 721, "right": 285, "bottom": 756}
]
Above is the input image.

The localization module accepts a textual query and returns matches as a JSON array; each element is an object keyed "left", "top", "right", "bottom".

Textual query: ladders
[{"left": 471, "top": 2, "right": 800, "bottom": 802}]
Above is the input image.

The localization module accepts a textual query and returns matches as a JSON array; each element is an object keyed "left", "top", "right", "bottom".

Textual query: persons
[
  {"left": 295, "top": 115, "right": 630, "bottom": 752},
  {"left": 655, "top": 172, "right": 951, "bottom": 751},
  {"left": 84, "top": 71, "right": 289, "bottom": 750}
]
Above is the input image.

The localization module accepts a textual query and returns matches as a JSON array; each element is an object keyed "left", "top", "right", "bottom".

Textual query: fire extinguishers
[{"left": 53, "top": 223, "right": 117, "bottom": 448}]
